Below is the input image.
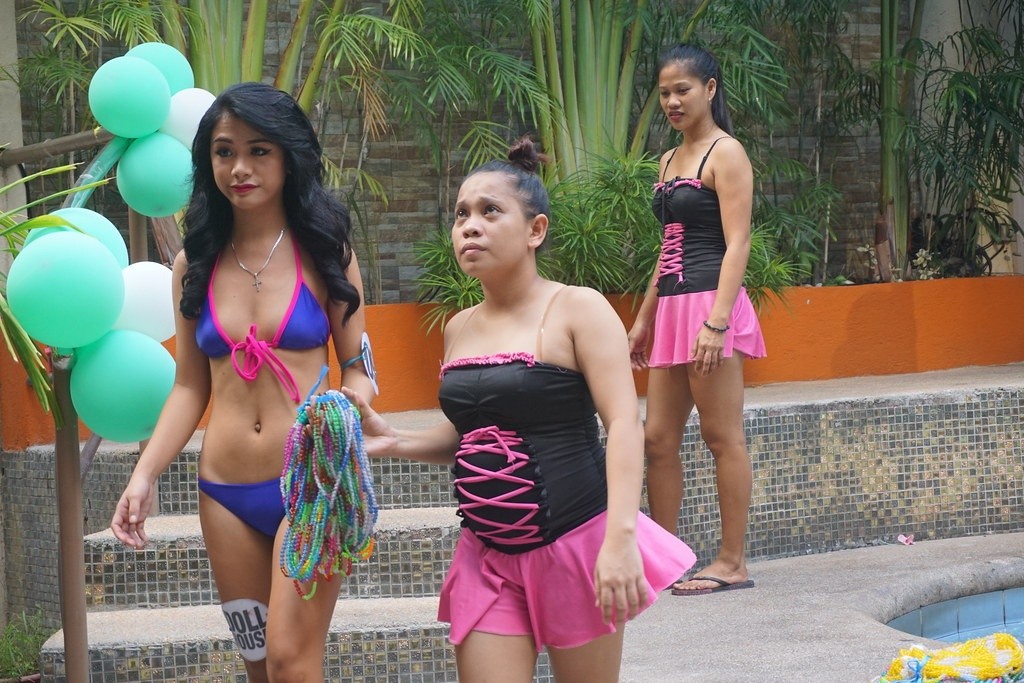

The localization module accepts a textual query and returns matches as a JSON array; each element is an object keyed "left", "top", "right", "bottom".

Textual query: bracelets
[{"left": 703, "top": 320, "right": 730, "bottom": 332}]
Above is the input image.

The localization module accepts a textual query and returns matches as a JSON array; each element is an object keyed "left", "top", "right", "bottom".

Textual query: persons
[
  {"left": 628, "top": 44, "right": 768, "bottom": 597},
  {"left": 305, "top": 136, "right": 697, "bottom": 683},
  {"left": 110, "top": 82, "right": 378, "bottom": 683}
]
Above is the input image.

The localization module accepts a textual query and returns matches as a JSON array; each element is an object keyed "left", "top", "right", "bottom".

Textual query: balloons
[
  {"left": 87, "top": 41, "right": 218, "bottom": 219},
  {"left": 4, "top": 207, "right": 176, "bottom": 444}
]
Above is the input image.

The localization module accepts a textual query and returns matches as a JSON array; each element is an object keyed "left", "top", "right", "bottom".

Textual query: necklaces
[
  {"left": 229, "top": 223, "right": 289, "bottom": 292},
  {"left": 280, "top": 389, "right": 379, "bottom": 602}
]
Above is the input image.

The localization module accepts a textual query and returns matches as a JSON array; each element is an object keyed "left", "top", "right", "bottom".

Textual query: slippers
[{"left": 671, "top": 577, "right": 754, "bottom": 596}]
